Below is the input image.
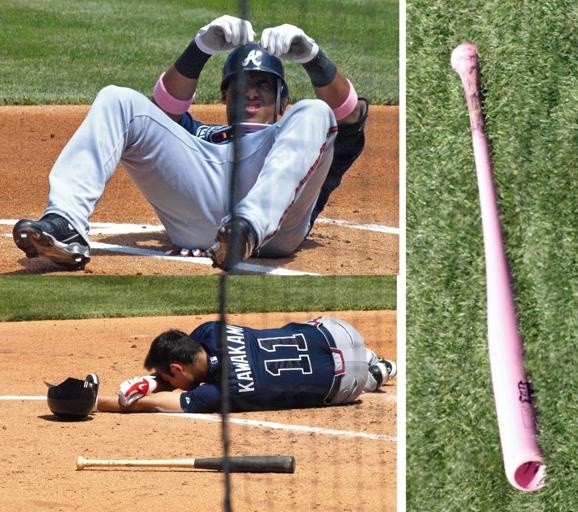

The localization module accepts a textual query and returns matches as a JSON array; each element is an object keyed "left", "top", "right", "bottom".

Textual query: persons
[
  {"left": 96, "top": 314, "right": 396, "bottom": 414},
  {"left": 13, "top": 14, "right": 370, "bottom": 272}
]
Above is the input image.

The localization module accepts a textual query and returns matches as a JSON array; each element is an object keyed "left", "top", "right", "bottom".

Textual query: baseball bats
[
  {"left": 452, "top": 44, "right": 547, "bottom": 492},
  {"left": 77, "top": 456, "right": 295, "bottom": 474}
]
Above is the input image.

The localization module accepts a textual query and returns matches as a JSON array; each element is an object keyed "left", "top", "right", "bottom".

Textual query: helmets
[
  {"left": 42, "top": 373, "right": 100, "bottom": 418},
  {"left": 220, "top": 40, "right": 289, "bottom": 100}
]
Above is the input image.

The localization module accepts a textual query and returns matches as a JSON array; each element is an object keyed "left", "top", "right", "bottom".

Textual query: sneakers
[
  {"left": 208, "top": 220, "right": 256, "bottom": 273},
  {"left": 12, "top": 211, "right": 91, "bottom": 272},
  {"left": 369, "top": 352, "right": 397, "bottom": 390}
]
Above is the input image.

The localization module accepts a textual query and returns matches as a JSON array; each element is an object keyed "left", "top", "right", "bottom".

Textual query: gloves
[
  {"left": 194, "top": 14, "right": 257, "bottom": 56},
  {"left": 260, "top": 22, "right": 318, "bottom": 65},
  {"left": 118, "top": 374, "right": 156, "bottom": 409}
]
